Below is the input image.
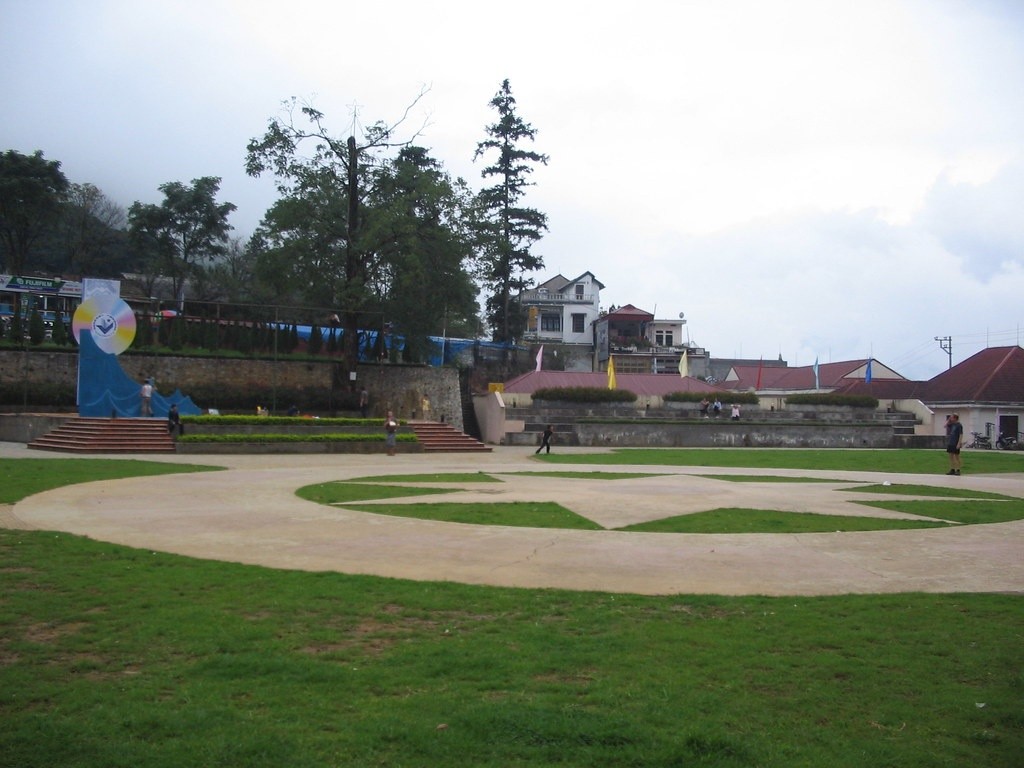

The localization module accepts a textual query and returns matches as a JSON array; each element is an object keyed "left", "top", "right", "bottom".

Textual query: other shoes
[
  {"left": 946, "top": 469, "right": 955, "bottom": 475},
  {"left": 955, "top": 468, "right": 961, "bottom": 476}
]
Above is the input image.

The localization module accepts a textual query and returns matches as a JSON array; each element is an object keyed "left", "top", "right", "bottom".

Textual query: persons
[
  {"left": 699, "top": 398, "right": 709, "bottom": 418},
  {"left": 167, "top": 404, "right": 184, "bottom": 435},
  {"left": 327, "top": 312, "right": 340, "bottom": 327},
  {"left": 731, "top": 403, "right": 741, "bottom": 420},
  {"left": 712, "top": 398, "right": 722, "bottom": 419},
  {"left": 943, "top": 413, "right": 962, "bottom": 475},
  {"left": 141, "top": 381, "right": 155, "bottom": 417},
  {"left": 536, "top": 425, "right": 554, "bottom": 454},
  {"left": 360, "top": 386, "right": 369, "bottom": 418},
  {"left": 385, "top": 411, "right": 400, "bottom": 456},
  {"left": 423, "top": 395, "right": 430, "bottom": 420}
]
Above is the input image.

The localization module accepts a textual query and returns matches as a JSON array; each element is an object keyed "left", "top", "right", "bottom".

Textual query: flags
[
  {"left": 813, "top": 358, "right": 820, "bottom": 390},
  {"left": 607, "top": 357, "right": 616, "bottom": 389},
  {"left": 756, "top": 359, "right": 762, "bottom": 390},
  {"left": 865, "top": 358, "right": 871, "bottom": 383},
  {"left": 678, "top": 350, "right": 688, "bottom": 377},
  {"left": 535, "top": 345, "right": 544, "bottom": 371}
]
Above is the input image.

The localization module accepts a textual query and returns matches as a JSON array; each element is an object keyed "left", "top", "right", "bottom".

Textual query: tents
[{"left": 601, "top": 304, "right": 654, "bottom": 322}]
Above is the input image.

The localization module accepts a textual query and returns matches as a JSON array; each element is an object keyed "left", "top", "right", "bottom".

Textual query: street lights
[
  {"left": 379, "top": 315, "right": 394, "bottom": 389},
  {"left": 150, "top": 297, "right": 160, "bottom": 363},
  {"left": 441, "top": 272, "right": 453, "bottom": 367},
  {"left": 20, "top": 294, "right": 44, "bottom": 351}
]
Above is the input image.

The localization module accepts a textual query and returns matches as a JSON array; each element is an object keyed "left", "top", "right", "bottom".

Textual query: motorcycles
[
  {"left": 995, "top": 431, "right": 1017, "bottom": 450},
  {"left": 971, "top": 432, "right": 991, "bottom": 449}
]
